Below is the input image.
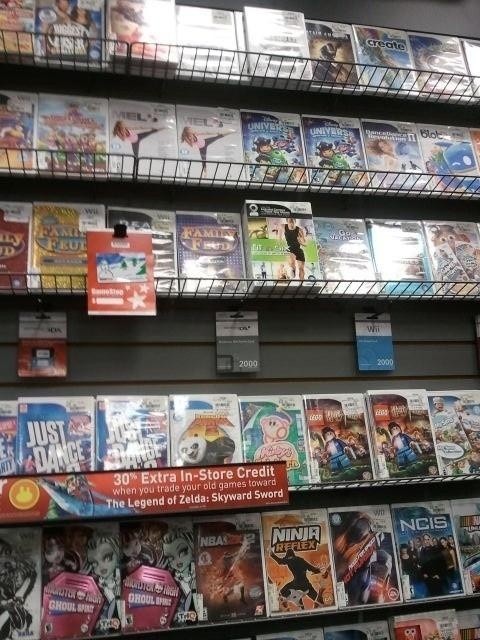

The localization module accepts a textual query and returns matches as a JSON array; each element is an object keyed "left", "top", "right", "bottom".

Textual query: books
[
  {"left": 1, "top": 200, "right": 480, "bottom": 300},
  {"left": 1, "top": 88, "right": 480, "bottom": 202},
  {"left": 1, "top": 1, "right": 479, "bottom": 103}
]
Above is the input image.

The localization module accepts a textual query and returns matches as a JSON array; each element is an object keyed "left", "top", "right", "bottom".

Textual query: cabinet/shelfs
[{"left": 0, "top": 0, "right": 480, "bottom": 640}]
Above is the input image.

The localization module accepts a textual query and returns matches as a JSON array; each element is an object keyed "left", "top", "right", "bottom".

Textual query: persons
[{"left": 396, "top": 532, "right": 461, "bottom": 599}]
